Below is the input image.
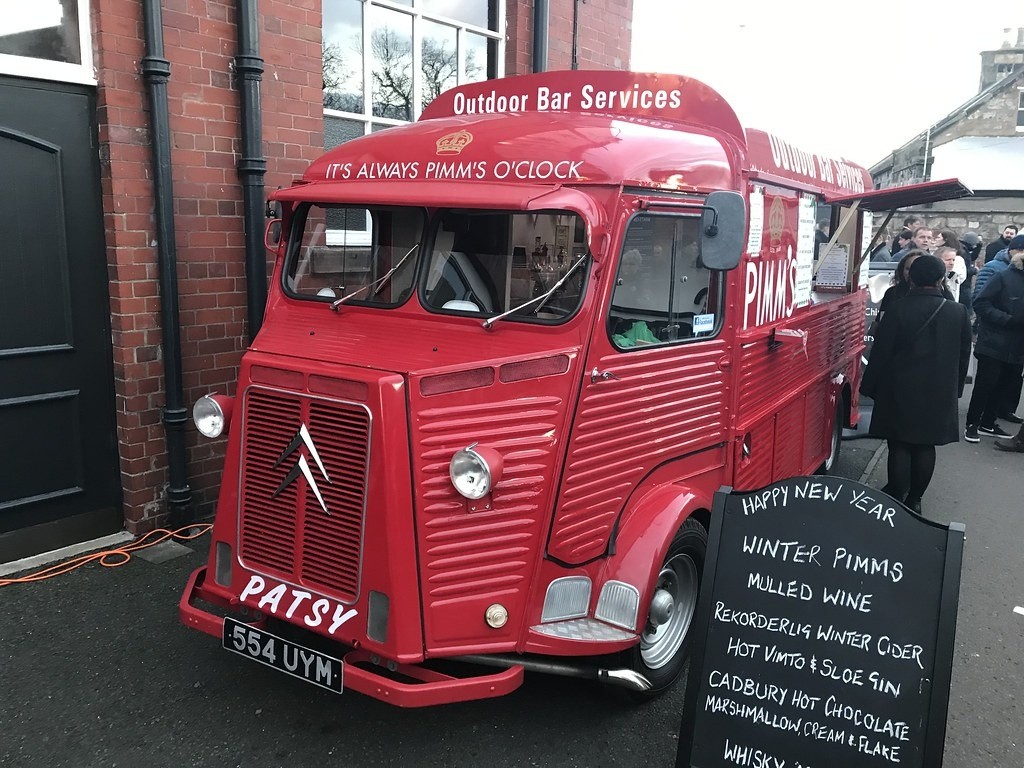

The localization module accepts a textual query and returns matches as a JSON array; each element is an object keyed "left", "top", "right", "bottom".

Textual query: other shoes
[
  {"left": 1000, "top": 412, "right": 1023, "bottom": 423},
  {"left": 906, "top": 502, "right": 921, "bottom": 515}
]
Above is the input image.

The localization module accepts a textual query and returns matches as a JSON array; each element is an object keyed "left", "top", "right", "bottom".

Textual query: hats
[
  {"left": 1008, "top": 236, "right": 1024, "bottom": 252},
  {"left": 964, "top": 231, "right": 979, "bottom": 246}
]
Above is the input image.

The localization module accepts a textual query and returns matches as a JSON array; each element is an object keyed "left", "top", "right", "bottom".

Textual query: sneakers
[{"left": 965, "top": 424, "right": 1013, "bottom": 443}]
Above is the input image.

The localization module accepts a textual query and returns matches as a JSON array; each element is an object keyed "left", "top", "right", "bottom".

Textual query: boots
[{"left": 996, "top": 422, "right": 1024, "bottom": 452}]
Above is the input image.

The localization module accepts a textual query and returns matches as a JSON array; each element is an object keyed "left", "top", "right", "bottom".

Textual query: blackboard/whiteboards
[{"left": 673, "top": 474, "right": 968, "bottom": 767}]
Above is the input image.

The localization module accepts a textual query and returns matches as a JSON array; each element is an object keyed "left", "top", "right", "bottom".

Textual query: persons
[
  {"left": 859, "top": 253, "right": 973, "bottom": 519},
  {"left": 971, "top": 246, "right": 1023, "bottom": 423},
  {"left": 611, "top": 235, "right": 699, "bottom": 310},
  {"left": 956, "top": 233, "right": 985, "bottom": 384},
  {"left": 964, "top": 253, "right": 1024, "bottom": 443},
  {"left": 932, "top": 247, "right": 961, "bottom": 307},
  {"left": 813, "top": 219, "right": 831, "bottom": 263},
  {"left": 870, "top": 252, "right": 958, "bottom": 498},
  {"left": 995, "top": 422, "right": 1024, "bottom": 452},
  {"left": 868, "top": 214, "right": 964, "bottom": 267},
  {"left": 984, "top": 225, "right": 1019, "bottom": 268}
]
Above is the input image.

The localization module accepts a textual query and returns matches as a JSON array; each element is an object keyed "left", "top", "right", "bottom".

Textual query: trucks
[{"left": 173, "top": 70, "right": 975, "bottom": 714}]
